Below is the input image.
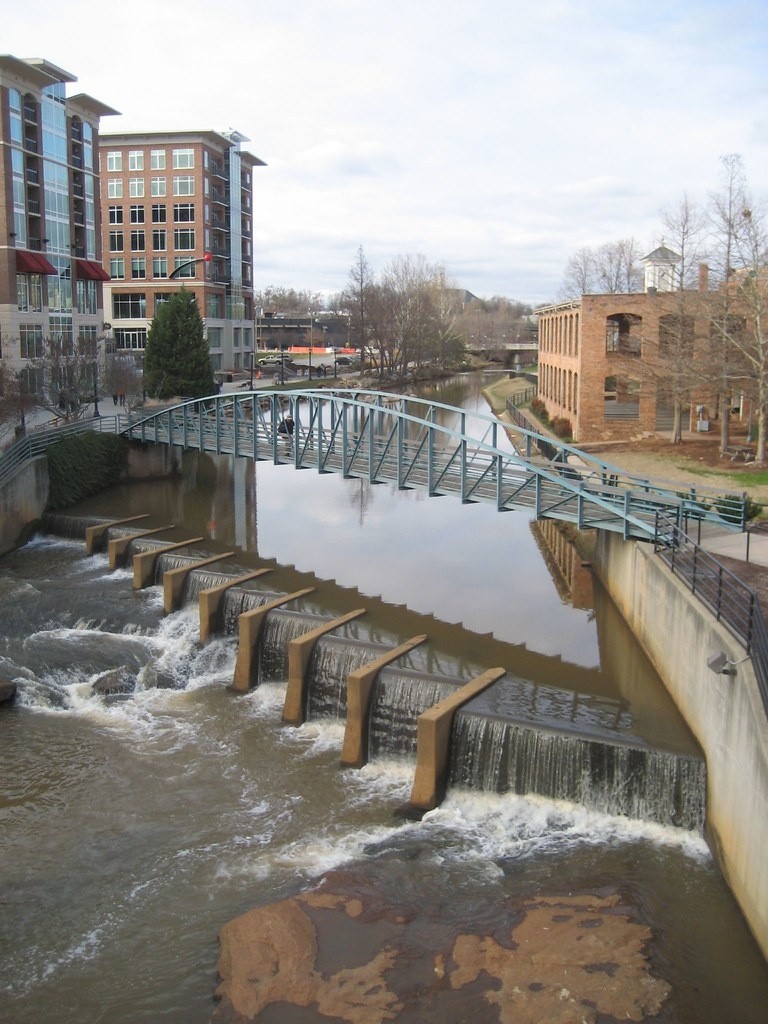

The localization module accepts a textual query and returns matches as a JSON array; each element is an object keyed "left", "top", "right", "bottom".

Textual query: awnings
[
  {"left": 59, "top": 266, "right": 70, "bottom": 280},
  {"left": 76, "top": 261, "right": 110, "bottom": 282},
  {"left": 17, "top": 251, "right": 58, "bottom": 275}
]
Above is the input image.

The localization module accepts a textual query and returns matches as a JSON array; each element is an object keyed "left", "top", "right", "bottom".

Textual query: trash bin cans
[{"left": 227, "top": 374, "right": 232, "bottom": 382}]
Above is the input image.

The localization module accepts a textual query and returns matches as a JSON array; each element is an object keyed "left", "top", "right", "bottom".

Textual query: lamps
[{"left": 707, "top": 651, "right": 737, "bottom": 680}]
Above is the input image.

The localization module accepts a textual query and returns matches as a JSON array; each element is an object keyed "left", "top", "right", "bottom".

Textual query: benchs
[{"left": 719, "top": 445, "right": 756, "bottom": 462}]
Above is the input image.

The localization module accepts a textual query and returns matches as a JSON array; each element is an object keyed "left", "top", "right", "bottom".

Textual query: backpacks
[{"left": 277, "top": 419, "right": 288, "bottom": 439}]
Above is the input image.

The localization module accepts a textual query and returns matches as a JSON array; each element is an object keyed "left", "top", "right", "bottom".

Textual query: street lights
[{"left": 306, "top": 307, "right": 317, "bottom": 381}]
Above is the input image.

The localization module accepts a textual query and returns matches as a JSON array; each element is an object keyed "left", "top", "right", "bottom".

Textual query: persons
[
  {"left": 316, "top": 363, "right": 327, "bottom": 378},
  {"left": 278, "top": 415, "right": 295, "bottom": 456},
  {"left": 58, "top": 386, "right": 126, "bottom": 411}
]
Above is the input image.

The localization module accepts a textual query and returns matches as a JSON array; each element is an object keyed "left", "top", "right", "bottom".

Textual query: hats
[{"left": 287, "top": 415, "right": 292, "bottom": 420}]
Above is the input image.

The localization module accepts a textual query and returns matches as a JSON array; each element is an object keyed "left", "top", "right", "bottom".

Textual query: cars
[
  {"left": 331, "top": 347, "right": 342, "bottom": 354},
  {"left": 334, "top": 356, "right": 356, "bottom": 366},
  {"left": 277, "top": 354, "right": 293, "bottom": 364}
]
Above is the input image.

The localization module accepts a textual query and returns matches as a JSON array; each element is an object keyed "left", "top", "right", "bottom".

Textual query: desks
[{"left": 726, "top": 446, "right": 753, "bottom": 463}]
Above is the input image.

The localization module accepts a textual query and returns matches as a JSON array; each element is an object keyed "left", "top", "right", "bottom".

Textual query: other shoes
[{"left": 285, "top": 454, "right": 291, "bottom": 457}]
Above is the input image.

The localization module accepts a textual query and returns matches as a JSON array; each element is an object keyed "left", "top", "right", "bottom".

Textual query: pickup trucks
[
  {"left": 359, "top": 346, "right": 380, "bottom": 354},
  {"left": 258, "top": 355, "right": 283, "bottom": 365}
]
